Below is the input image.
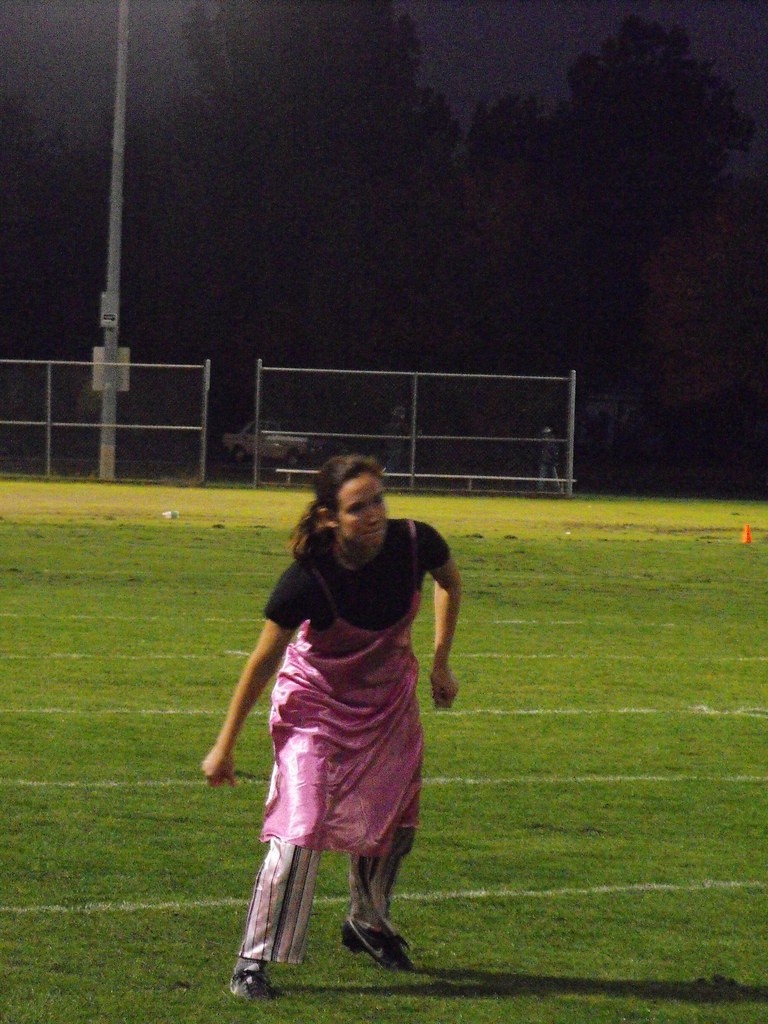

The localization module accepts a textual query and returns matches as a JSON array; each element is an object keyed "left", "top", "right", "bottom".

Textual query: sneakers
[
  {"left": 340, "top": 919, "right": 415, "bottom": 975},
  {"left": 230, "top": 962, "right": 276, "bottom": 1002}
]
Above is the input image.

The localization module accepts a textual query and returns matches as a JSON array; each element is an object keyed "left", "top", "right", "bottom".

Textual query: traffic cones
[{"left": 742, "top": 524, "right": 751, "bottom": 543}]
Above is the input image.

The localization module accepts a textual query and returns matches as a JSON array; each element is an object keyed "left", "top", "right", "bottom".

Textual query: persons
[
  {"left": 381, "top": 407, "right": 418, "bottom": 481},
  {"left": 537, "top": 428, "right": 562, "bottom": 492},
  {"left": 202, "top": 456, "right": 460, "bottom": 998}
]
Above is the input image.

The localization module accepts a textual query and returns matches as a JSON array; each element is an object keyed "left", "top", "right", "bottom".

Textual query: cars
[{"left": 224, "top": 416, "right": 350, "bottom": 467}]
